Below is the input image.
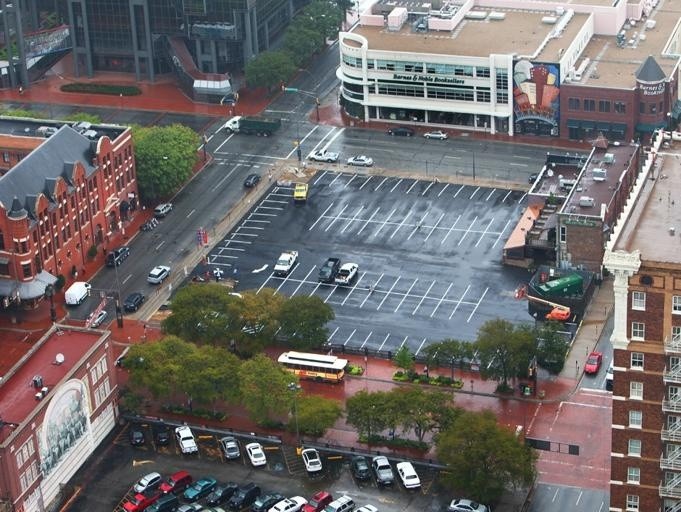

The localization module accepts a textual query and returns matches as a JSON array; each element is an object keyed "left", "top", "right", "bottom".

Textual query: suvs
[
  {"left": 105, "top": 246, "right": 130, "bottom": 266},
  {"left": 372, "top": 455, "right": 395, "bottom": 486},
  {"left": 350, "top": 455, "right": 372, "bottom": 481},
  {"left": 153, "top": 203, "right": 172, "bottom": 217},
  {"left": 302, "top": 448, "right": 322, "bottom": 472},
  {"left": 147, "top": 265, "right": 171, "bottom": 284},
  {"left": 123, "top": 471, "right": 378, "bottom": 512},
  {"left": 397, "top": 461, "right": 422, "bottom": 488}
]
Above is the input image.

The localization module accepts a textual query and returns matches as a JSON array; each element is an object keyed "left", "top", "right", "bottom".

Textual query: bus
[{"left": 278, "top": 351, "right": 348, "bottom": 384}]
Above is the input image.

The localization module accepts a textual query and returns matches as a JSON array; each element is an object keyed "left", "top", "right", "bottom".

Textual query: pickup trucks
[
  {"left": 174, "top": 425, "right": 199, "bottom": 453},
  {"left": 275, "top": 250, "right": 299, "bottom": 276},
  {"left": 309, "top": 148, "right": 339, "bottom": 163},
  {"left": 335, "top": 263, "right": 359, "bottom": 285},
  {"left": 319, "top": 258, "right": 341, "bottom": 282}
]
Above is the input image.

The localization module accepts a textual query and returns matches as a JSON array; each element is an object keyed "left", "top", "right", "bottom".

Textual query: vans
[
  {"left": 65, "top": 282, "right": 92, "bottom": 306},
  {"left": 606, "top": 360, "right": 613, "bottom": 387}
]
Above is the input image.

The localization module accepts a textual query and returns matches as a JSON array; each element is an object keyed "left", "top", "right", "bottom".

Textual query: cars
[
  {"left": 388, "top": 127, "right": 415, "bottom": 137},
  {"left": 152, "top": 424, "right": 171, "bottom": 447},
  {"left": 423, "top": 130, "right": 450, "bottom": 140},
  {"left": 584, "top": 350, "right": 603, "bottom": 374},
  {"left": 218, "top": 436, "right": 241, "bottom": 460},
  {"left": 294, "top": 183, "right": 309, "bottom": 201},
  {"left": 85, "top": 310, "right": 108, "bottom": 327},
  {"left": 245, "top": 442, "right": 267, "bottom": 467},
  {"left": 529, "top": 172, "right": 540, "bottom": 184},
  {"left": 124, "top": 292, "right": 146, "bottom": 313},
  {"left": 348, "top": 154, "right": 374, "bottom": 167},
  {"left": 244, "top": 174, "right": 262, "bottom": 187},
  {"left": 449, "top": 498, "right": 488, "bottom": 512},
  {"left": 128, "top": 422, "right": 146, "bottom": 447}
]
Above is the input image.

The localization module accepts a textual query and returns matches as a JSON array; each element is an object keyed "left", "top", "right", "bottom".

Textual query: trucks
[{"left": 224, "top": 115, "right": 282, "bottom": 137}]
